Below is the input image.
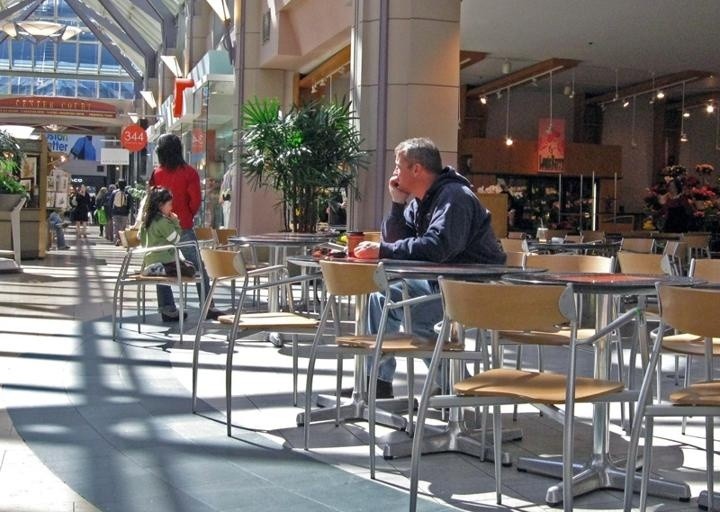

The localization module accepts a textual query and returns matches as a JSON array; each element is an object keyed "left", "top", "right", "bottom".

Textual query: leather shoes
[
  {"left": 332, "top": 378, "right": 395, "bottom": 405},
  {"left": 427, "top": 383, "right": 474, "bottom": 409}
]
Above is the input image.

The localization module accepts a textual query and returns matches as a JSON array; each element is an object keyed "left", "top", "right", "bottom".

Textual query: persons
[
  {"left": 148, "top": 132, "right": 225, "bottom": 322},
  {"left": 47, "top": 179, "right": 133, "bottom": 251},
  {"left": 335, "top": 136, "right": 509, "bottom": 412},
  {"left": 137, "top": 183, "right": 198, "bottom": 279}
]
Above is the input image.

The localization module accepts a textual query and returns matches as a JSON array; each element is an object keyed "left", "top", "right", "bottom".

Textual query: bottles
[{"left": 347, "top": 231, "right": 365, "bottom": 258}]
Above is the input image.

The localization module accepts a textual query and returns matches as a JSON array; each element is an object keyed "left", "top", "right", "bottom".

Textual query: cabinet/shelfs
[
  {"left": 465, "top": 168, "right": 624, "bottom": 230},
  {"left": 473, "top": 191, "right": 511, "bottom": 239}
]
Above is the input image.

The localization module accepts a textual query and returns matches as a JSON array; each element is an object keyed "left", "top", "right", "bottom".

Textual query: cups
[
  {"left": 364, "top": 232, "right": 382, "bottom": 243},
  {"left": 537, "top": 227, "right": 549, "bottom": 244}
]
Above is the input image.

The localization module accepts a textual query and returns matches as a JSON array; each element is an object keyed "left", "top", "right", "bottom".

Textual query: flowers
[{"left": 640, "top": 159, "right": 719, "bottom": 230}]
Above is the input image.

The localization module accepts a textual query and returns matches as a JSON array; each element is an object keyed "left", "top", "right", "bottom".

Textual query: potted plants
[{"left": 218, "top": 92, "right": 378, "bottom": 286}]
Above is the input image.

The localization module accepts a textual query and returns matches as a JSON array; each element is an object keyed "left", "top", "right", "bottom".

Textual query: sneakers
[
  {"left": 206, "top": 309, "right": 226, "bottom": 321},
  {"left": 161, "top": 309, "right": 188, "bottom": 323},
  {"left": 77, "top": 233, "right": 121, "bottom": 247},
  {"left": 142, "top": 261, "right": 164, "bottom": 276}
]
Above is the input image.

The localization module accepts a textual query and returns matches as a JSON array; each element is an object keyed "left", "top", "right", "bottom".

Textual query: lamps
[
  {"left": 126, "top": 111, "right": 143, "bottom": 125},
  {"left": 0, "top": 1, "right": 83, "bottom": 46},
  {"left": 202, "top": 0, "right": 240, "bottom": 64},
  {"left": 462, "top": 53, "right": 719, "bottom": 146},
  {"left": 306, "top": 61, "right": 351, "bottom": 95},
  {"left": 156, "top": 53, "right": 186, "bottom": 83},
  {"left": 139, "top": 89, "right": 158, "bottom": 114}
]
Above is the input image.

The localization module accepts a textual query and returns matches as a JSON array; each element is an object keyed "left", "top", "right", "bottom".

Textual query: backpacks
[{"left": 113, "top": 191, "right": 127, "bottom": 208}]
